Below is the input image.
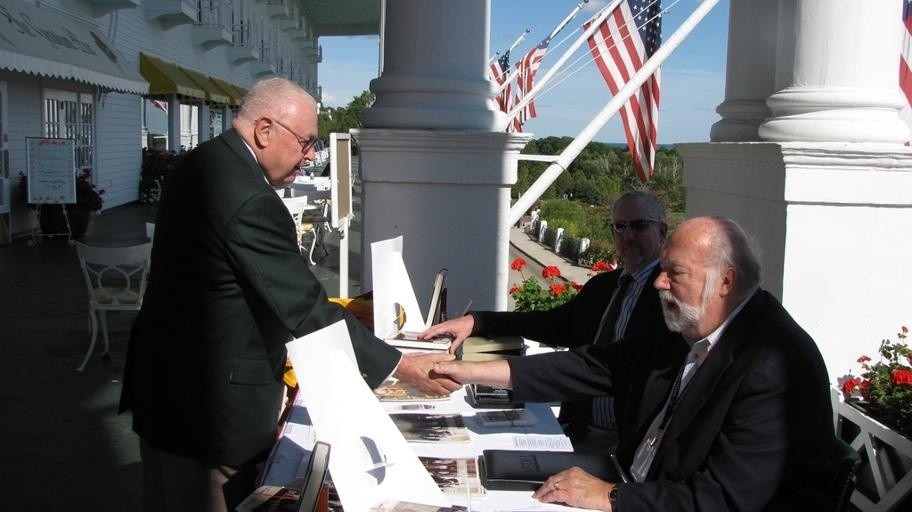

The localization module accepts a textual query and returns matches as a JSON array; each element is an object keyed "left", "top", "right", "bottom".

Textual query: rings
[{"left": 555, "top": 480, "right": 561, "bottom": 490}]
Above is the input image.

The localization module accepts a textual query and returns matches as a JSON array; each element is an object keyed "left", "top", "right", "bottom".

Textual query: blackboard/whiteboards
[{"left": 26, "top": 137, "right": 76, "bottom": 204}]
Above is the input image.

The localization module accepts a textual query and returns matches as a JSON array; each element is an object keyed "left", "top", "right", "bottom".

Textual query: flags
[
  {"left": 901, "top": 0, "right": 912, "bottom": 150},
  {"left": 515, "top": 35, "right": 550, "bottom": 126},
  {"left": 581, "top": 1, "right": 664, "bottom": 185},
  {"left": 491, "top": 51, "right": 523, "bottom": 133}
]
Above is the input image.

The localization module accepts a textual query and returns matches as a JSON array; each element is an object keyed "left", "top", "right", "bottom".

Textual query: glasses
[
  {"left": 255, "top": 116, "right": 318, "bottom": 153},
  {"left": 611, "top": 218, "right": 662, "bottom": 234}
]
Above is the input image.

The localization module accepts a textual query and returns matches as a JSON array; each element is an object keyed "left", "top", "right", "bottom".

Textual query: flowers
[{"left": 19, "top": 168, "right": 105, "bottom": 210}]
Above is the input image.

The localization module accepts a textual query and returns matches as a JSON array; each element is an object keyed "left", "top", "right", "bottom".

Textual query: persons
[
  {"left": 422, "top": 192, "right": 680, "bottom": 460},
  {"left": 121, "top": 75, "right": 461, "bottom": 510},
  {"left": 431, "top": 215, "right": 830, "bottom": 510}
]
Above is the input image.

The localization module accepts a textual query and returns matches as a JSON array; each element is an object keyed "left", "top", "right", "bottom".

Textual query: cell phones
[{"left": 477, "top": 410, "right": 538, "bottom": 427}]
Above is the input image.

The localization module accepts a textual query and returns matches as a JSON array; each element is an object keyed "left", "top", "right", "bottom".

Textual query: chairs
[
  {"left": 814, "top": 437, "right": 863, "bottom": 511},
  {"left": 72, "top": 239, "right": 150, "bottom": 371},
  {"left": 274, "top": 176, "right": 333, "bottom": 266}
]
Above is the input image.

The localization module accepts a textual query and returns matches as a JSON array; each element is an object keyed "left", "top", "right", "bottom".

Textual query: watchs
[{"left": 609, "top": 482, "right": 618, "bottom": 511}]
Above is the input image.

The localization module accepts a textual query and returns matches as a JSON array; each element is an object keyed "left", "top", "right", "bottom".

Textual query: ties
[{"left": 596, "top": 274, "right": 632, "bottom": 343}]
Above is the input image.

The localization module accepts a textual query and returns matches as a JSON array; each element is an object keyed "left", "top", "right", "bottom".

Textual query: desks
[{"left": 255, "top": 348, "right": 603, "bottom": 512}]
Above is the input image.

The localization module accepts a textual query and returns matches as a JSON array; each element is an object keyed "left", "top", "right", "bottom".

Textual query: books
[
  {"left": 296, "top": 441, "right": 331, "bottom": 510},
  {"left": 234, "top": 487, "right": 300, "bottom": 511},
  {"left": 424, "top": 267, "right": 449, "bottom": 330},
  {"left": 382, "top": 318, "right": 538, "bottom": 445},
  {"left": 478, "top": 441, "right": 623, "bottom": 495}
]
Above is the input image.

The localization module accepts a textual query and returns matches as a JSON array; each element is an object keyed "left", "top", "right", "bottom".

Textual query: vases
[{"left": 38, "top": 207, "right": 93, "bottom": 238}]
